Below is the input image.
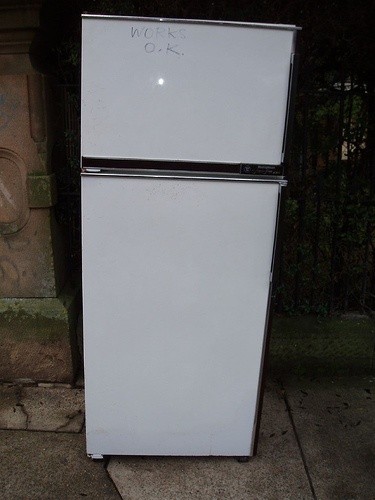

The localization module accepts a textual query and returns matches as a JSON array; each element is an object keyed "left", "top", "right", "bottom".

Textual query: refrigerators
[{"left": 79, "top": 12, "right": 304, "bottom": 463}]
